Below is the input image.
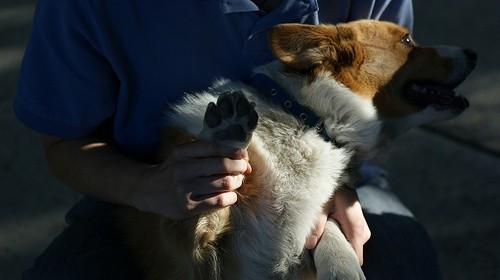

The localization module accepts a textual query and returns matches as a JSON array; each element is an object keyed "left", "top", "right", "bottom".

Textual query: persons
[{"left": 11, "top": 0, "right": 443, "bottom": 280}]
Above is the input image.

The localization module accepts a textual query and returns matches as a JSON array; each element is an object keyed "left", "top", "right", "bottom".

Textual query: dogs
[{"left": 112, "top": 18, "right": 477, "bottom": 280}]
